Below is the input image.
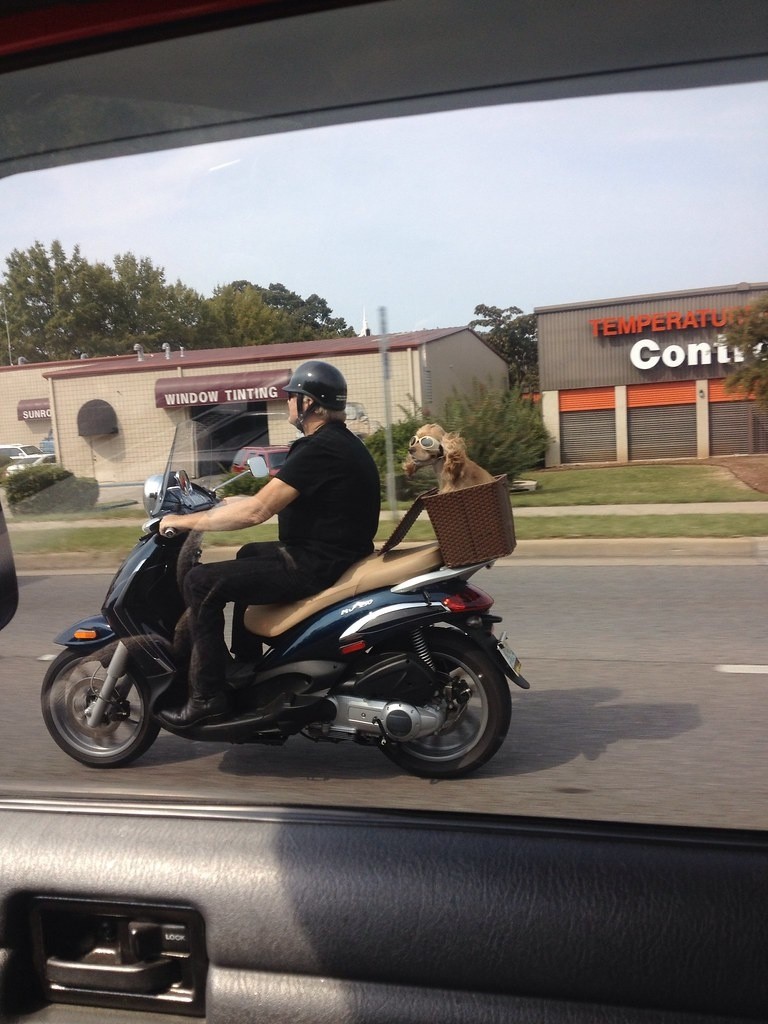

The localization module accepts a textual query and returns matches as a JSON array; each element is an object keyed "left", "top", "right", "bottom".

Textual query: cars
[{"left": 0, "top": 443, "right": 57, "bottom": 479}]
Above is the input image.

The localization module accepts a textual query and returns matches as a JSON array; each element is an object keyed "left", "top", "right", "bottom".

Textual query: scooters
[{"left": 39, "top": 420, "right": 530, "bottom": 782}]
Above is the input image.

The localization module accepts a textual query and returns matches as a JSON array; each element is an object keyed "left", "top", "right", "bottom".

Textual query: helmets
[{"left": 281, "top": 361, "right": 347, "bottom": 410}]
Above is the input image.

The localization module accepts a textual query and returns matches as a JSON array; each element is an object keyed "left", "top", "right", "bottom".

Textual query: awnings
[{"left": 154, "top": 369, "right": 292, "bottom": 408}]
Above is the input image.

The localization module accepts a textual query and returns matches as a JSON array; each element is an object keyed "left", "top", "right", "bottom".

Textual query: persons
[{"left": 158, "top": 359, "right": 382, "bottom": 731}]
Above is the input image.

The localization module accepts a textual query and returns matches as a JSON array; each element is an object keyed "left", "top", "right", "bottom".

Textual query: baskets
[{"left": 376, "top": 473, "right": 517, "bottom": 568}]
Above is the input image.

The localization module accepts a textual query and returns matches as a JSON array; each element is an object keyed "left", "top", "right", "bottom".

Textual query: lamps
[{"left": 700, "top": 389, "right": 703, "bottom": 398}]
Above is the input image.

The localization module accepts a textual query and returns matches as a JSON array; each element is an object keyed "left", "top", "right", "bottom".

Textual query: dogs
[{"left": 400, "top": 421, "right": 499, "bottom": 496}]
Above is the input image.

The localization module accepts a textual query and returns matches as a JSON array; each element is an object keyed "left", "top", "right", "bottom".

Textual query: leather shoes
[{"left": 157, "top": 688, "right": 233, "bottom": 729}]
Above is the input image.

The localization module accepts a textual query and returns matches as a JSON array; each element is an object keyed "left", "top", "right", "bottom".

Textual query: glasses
[
  {"left": 286, "top": 392, "right": 306, "bottom": 401},
  {"left": 409, "top": 436, "right": 441, "bottom": 450}
]
